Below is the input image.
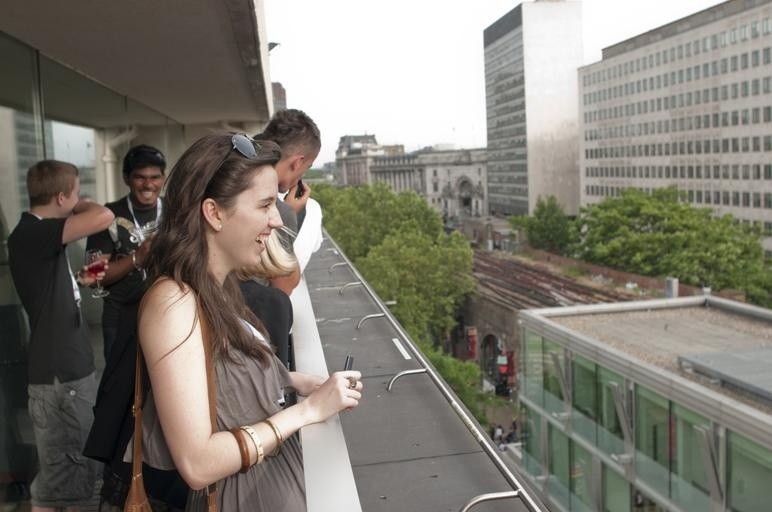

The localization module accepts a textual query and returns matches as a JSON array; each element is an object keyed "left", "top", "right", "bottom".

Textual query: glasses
[
  {"left": 128, "top": 151, "right": 161, "bottom": 160},
  {"left": 224, "top": 133, "right": 262, "bottom": 160}
]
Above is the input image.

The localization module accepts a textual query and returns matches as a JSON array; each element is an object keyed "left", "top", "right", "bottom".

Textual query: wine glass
[{"left": 85, "top": 248, "right": 111, "bottom": 299}]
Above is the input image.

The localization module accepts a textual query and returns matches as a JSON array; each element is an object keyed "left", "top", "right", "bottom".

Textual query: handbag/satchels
[{"left": 123, "top": 476, "right": 219, "bottom": 512}]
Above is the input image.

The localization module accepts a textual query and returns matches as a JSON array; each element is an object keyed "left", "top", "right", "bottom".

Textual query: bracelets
[
  {"left": 74, "top": 270, "right": 89, "bottom": 289},
  {"left": 130, "top": 251, "right": 143, "bottom": 273},
  {"left": 240, "top": 425, "right": 265, "bottom": 467},
  {"left": 228, "top": 426, "right": 251, "bottom": 475},
  {"left": 262, "top": 416, "right": 284, "bottom": 459}
]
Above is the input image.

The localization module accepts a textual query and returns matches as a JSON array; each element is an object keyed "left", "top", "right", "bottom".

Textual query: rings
[{"left": 345, "top": 376, "right": 357, "bottom": 392}]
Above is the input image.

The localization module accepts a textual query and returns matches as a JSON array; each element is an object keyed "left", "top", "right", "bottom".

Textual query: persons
[
  {"left": 133, "top": 131, "right": 365, "bottom": 512},
  {"left": 82, "top": 143, "right": 174, "bottom": 367},
  {"left": 6, "top": 157, "right": 118, "bottom": 512},
  {"left": 250, "top": 132, "right": 325, "bottom": 277},
  {"left": 251, "top": 108, "right": 326, "bottom": 299},
  {"left": 224, "top": 217, "right": 293, "bottom": 380},
  {"left": 633, "top": 488, "right": 646, "bottom": 512},
  {"left": 493, "top": 423, "right": 502, "bottom": 441}
]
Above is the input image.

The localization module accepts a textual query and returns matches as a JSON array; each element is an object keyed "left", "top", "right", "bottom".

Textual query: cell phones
[{"left": 343, "top": 356, "right": 354, "bottom": 371}]
